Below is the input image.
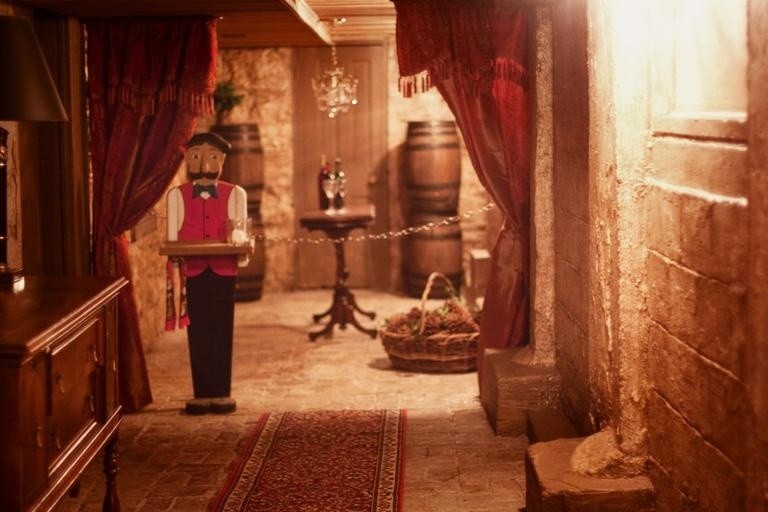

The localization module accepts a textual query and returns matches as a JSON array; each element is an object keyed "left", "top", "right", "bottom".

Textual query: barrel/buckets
[
  {"left": 232, "top": 210, "right": 267, "bottom": 301},
  {"left": 212, "top": 121, "right": 263, "bottom": 211},
  {"left": 406, "top": 212, "right": 465, "bottom": 299},
  {"left": 405, "top": 121, "right": 462, "bottom": 214}
]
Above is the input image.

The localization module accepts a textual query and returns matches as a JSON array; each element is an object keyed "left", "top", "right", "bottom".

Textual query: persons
[{"left": 163, "top": 130, "right": 256, "bottom": 414}]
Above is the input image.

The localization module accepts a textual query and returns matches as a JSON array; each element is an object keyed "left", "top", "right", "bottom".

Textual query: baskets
[{"left": 378, "top": 272, "right": 480, "bottom": 372}]
